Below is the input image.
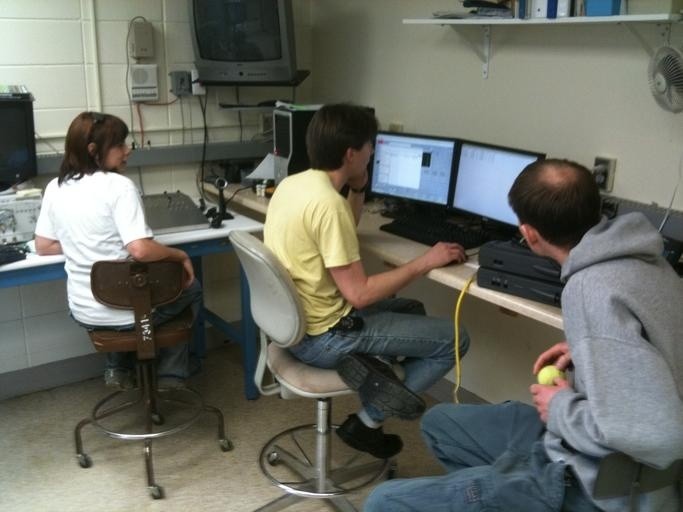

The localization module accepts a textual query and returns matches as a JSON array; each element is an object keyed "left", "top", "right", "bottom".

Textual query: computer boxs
[{"left": 272, "top": 109, "right": 318, "bottom": 185}]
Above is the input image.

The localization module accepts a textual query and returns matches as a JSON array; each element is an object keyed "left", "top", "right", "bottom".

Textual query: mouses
[{"left": 444, "top": 253, "right": 469, "bottom": 266}]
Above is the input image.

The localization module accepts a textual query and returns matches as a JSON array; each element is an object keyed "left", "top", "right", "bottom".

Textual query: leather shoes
[
  {"left": 334, "top": 351, "right": 428, "bottom": 421},
  {"left": 336, "top": 412, "right": 404, "bottom": 459}
]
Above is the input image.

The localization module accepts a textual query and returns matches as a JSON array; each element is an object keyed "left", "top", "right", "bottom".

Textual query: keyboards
[{"left": 381, "top": 211, "right": 495, "bottom": 249}]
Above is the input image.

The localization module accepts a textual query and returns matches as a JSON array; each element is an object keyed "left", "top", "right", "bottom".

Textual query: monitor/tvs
[
  {"left": 0, "top": 101, "right": 37, "bottom": 193},
  {"left": 189, "top": 0, "right": 297, "bottom": 82},
  {"left": 452, "top": 141, "right": 546, "bottom": 235},
  {"left": 367, "top": 131, "right": 458, "bottom": 217}
]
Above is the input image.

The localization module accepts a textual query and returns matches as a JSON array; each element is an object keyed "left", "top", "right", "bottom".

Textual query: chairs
[
  {"left": 73, "top": 260, "right": 233, "bottom": 500},
  {"left": 229, "top": 230, "right": 405, "bottom": 512}
]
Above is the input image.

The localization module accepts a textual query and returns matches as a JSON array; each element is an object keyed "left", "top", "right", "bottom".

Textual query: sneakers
[
  {"left": 102, "top": 368, "right": 140, "bottom": 393},
  {"left": 154, "top": 375, "right": 194, "bottom": 395}
]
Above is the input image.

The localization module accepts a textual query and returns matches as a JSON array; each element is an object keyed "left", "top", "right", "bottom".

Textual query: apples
[{"left": 537, "top": 364, "right": 565, "bottom": 386}]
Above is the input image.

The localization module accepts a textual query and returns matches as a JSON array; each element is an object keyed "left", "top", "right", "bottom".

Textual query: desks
[
  {"left": 0, "top": 197, "right": 265, "bottom": 401},
  {"left": 198, "top": 174, "right": 564, "bottom": 333}
]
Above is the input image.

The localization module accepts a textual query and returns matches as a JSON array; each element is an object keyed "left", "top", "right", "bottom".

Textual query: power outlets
[{"left": 593, "top": 155, "right": 616, "bottom": 192}]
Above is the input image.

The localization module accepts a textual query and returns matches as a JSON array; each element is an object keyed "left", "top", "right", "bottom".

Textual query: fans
[{"left": 647, "top": 43, "right": 683, "bottom": 113}]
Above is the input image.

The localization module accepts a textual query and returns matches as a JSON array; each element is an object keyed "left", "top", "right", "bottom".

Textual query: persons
[
  {"left": 261, "top": 102, "right": 472, "bottom": 460},
  {"left": 33, "top": 109, "right": 210, "bottom": 391},
  {"left": 361, "top": 157, "right": 682, "bottom": 511}
]
